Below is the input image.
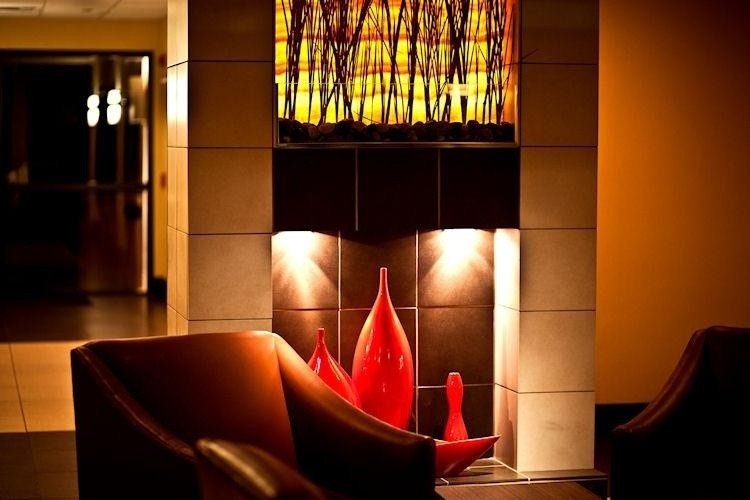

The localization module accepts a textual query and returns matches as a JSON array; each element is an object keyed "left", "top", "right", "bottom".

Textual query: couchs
[
  {"left": 70, "top": 331, "right": 435, "bottom": 500},
  {"left": 609, "top": 325, "right": 750, "bottom": 500}
]
